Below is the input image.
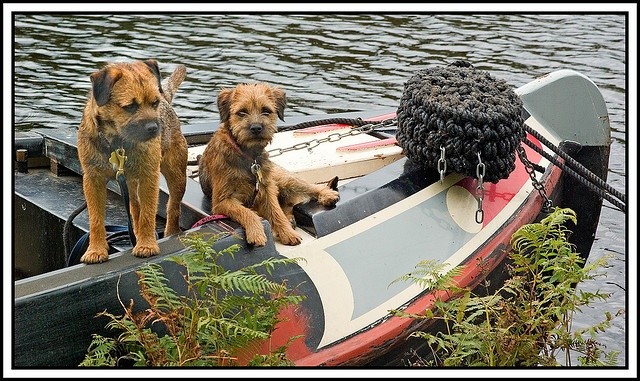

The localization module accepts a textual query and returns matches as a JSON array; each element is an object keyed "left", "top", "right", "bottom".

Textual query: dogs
[
  {"left": 196, "top": 81, "right": 338, "bottom": 247},
  {"left": 78, "top": 59, "right": 188, "bottom": 264}
]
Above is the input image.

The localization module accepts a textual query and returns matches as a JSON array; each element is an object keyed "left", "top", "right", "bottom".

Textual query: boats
[{"left": 12, "top": 66, "right": 611, "bottom": 369}]
